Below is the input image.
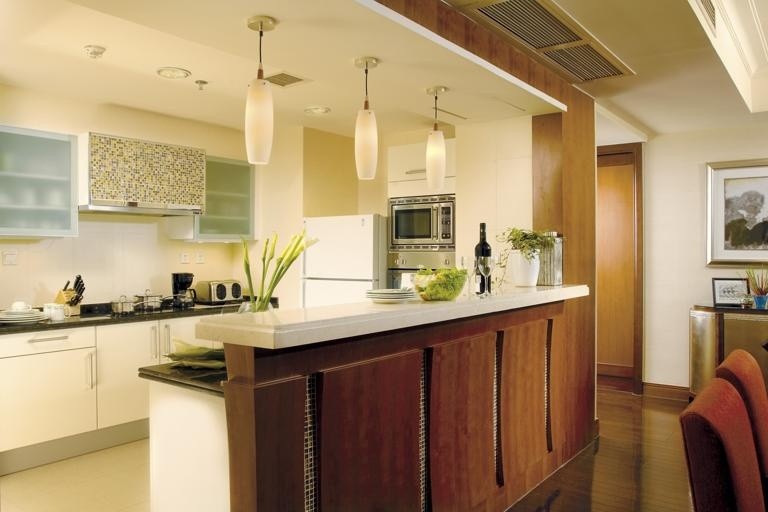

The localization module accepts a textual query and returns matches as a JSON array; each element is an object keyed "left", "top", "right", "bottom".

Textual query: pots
[
  {"left": 133, "top": 289, "right": 181, "bottom": 310},
  {"left": 111, "top": 295, "right": 163, "bottom": 315}
]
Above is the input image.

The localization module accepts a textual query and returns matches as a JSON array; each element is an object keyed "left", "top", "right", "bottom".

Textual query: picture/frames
[
  {"left": 707, "top": 158, "right": 768, "bottom": 268},
  {"left": 712, "top": 278, "right": 750, "bottom": 307}
]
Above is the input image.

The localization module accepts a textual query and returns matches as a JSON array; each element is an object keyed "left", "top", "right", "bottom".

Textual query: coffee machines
[{"left": 170, "top": 272, "right": 195, "bottom": 307}]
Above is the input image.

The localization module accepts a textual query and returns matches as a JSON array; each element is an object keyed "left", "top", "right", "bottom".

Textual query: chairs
[{"left": 679, "top": 348, "right": 768, "bottom": 512}]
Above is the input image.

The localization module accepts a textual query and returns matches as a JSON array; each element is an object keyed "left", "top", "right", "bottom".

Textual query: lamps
[
  {"left": 244, "top": 16, "right": 276, "bottom": 164},
  {"left": 354, "top": 56, "right": 379, "bottom": 180},
  {"left": 425, "top": 86, "right": 446, "bottom": 188}
]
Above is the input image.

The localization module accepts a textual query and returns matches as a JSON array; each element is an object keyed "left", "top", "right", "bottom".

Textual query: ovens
[{"left": 389, "top": 251, "right": 456, "bottom": 292}]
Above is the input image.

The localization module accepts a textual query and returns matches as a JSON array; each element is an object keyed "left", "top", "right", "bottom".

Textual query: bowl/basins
[{"left": 411, "top": 272, "right": 467, "bottom": 302}]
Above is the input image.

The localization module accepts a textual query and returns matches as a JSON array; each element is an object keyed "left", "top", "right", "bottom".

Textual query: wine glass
[
  {"left": 493, "top": 256, "right": 506, "bottom": 296},
  {"left": 462, "top": 255, "right": 479, "bottom": 296},
  {"left": 478, "top": 256, "right": 493, "bottom": 297}
]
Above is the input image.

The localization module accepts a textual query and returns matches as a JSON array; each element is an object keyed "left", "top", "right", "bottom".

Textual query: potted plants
[
  {"left": 495, "top": 227, "right": 567, "bottom": 287},
  {"left": 742, "top": 263, "right": 768, "bottom": 309}
]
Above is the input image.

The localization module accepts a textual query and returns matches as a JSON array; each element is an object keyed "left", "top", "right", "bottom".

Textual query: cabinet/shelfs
[
  {"left": 0, "top": 326, "right": 97, "bottom": 475},
  {"left": 166, "top": 154, "right": 256, "bottom": 245},
  {"left": 95, "top": 313, "right": 214, "bottom": 450},
  {"left": 1, "top": 126, "right": 79, "bottom": 240}
]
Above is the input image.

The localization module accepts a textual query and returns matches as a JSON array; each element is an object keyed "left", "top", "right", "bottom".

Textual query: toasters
[{"left": 196, "top": 279, "right": 243, "bottom": 304}]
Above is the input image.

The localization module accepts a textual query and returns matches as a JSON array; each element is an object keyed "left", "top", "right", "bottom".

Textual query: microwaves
[{"left": 392, "top": 202, "right": 454, "bottom": 245}]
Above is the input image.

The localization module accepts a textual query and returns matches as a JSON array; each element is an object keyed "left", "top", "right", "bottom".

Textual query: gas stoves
[{"left": 107, "top": 306, "right": 177, "bottom": 316}]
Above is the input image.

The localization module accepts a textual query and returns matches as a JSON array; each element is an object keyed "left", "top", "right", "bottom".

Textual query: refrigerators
[{"left": 303, "top": 214, "right": 387, "bottom": 308}]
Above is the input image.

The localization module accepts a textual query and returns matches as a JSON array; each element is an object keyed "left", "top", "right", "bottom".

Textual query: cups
[
  {"left": 754, "top": 295, "right": 767, "bottom": 308},
  {"left": 44, "top": 303, "right": 57, "bottom": 319},
  {"left": 52, "top": 304, "right": 71, "bottom": 320},
  {"left": 12, "top": 302, "right": 32, "bottom": 312}
]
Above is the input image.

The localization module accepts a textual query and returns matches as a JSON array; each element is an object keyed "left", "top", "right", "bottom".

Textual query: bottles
[{"left": 475, "top": 223, "right": 492, "bottom": 295}]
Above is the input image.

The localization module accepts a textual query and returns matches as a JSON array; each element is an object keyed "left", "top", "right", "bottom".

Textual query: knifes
[{"left": 54, "top": 274, "right": 85, "bottom": 316}]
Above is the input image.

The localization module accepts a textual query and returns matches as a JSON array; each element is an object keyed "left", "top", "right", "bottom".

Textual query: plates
[
  {"left": 365, "top": 288, "right": 414, "bottom": 303},
  {"left": 0, "top": 309, "right": 50, "bottom": 324}
]
